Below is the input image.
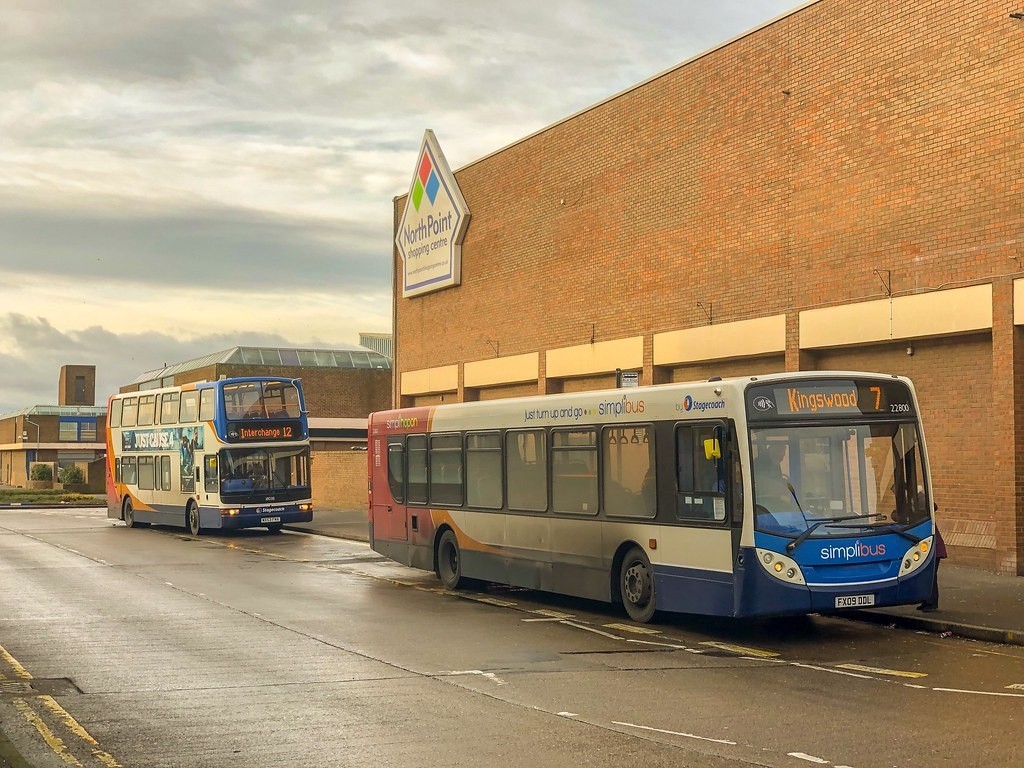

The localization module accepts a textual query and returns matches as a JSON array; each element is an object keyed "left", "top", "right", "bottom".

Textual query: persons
[
  {"left": 753, "top": 443, "right": 788, "bottom": 478},
  {"left": 182, "top": 427, "right": 199, "bottom": 472},
  {"left": 713, "top": 466, "right": 742, "bottom": 498}
]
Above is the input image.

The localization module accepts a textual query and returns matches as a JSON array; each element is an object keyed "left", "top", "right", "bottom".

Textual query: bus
[
  {"left": 367, "top": 369, "right": 936, "bottom": 624},
  {"left": 106, "top": 377, "right": 314, "bottom": 537}
]
